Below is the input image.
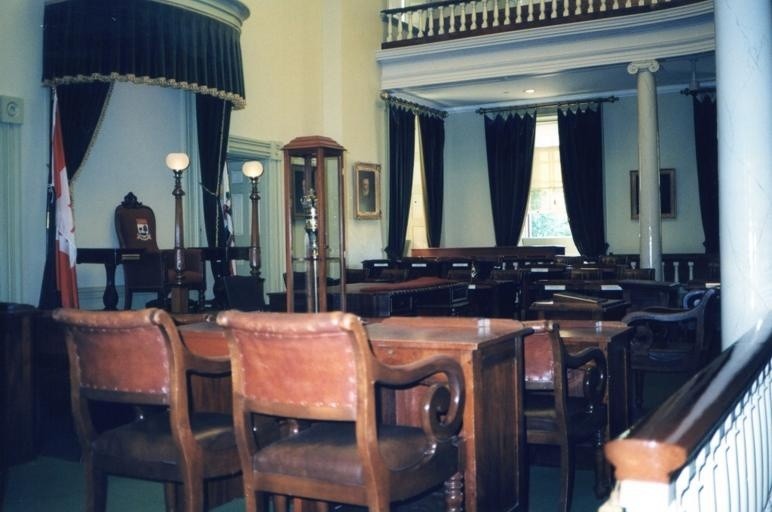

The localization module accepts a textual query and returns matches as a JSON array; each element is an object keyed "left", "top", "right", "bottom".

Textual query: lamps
[
  {"left": 241, "top": 160, "right": 264, "bottom": 275},
  {"left": 166, "top": 152, "right": 190, "bottom": 311}
]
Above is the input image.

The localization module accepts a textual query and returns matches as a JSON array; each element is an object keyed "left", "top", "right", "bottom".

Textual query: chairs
[
  {"left": 114, "top": 191, "right": 206, "bottom": 310},
  {"left": 0, "top": 303, "right": 36, "bottom": 472}
]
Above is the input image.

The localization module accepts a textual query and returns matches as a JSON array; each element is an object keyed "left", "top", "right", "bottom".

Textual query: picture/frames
[
  {"left": 352, "top": 162, "right": 382, "bottom": 221},
  {"left": 630, "top": 169, "right": 678, "bottom": 221},
  {"left": 292, "top": 164, "right": 317, "bottom": 221}
]
